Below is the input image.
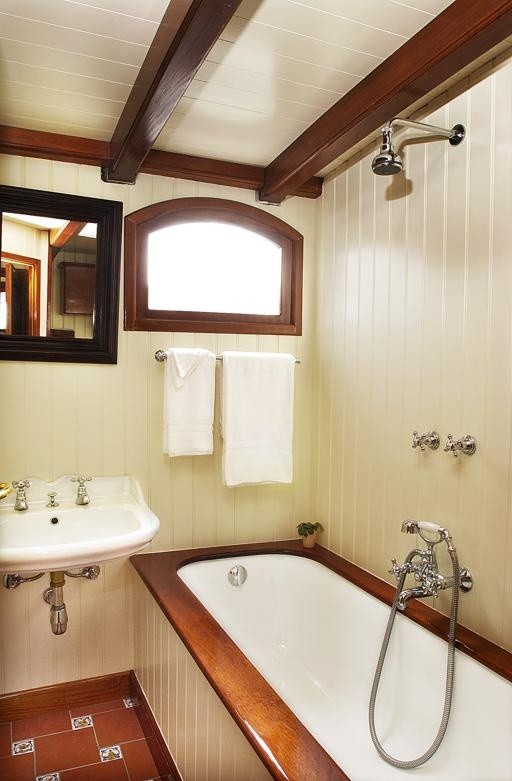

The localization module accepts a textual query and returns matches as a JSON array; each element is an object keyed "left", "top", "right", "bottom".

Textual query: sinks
[{"left": 0, "top": 475, "right": 161, "bottom": 572}]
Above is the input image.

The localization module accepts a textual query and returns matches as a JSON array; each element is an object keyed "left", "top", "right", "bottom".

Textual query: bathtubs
[{"left": 130, "top": 538, "right": 512, "bottom": 781}]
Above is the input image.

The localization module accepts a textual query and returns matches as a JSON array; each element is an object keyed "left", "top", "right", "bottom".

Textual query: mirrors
[{"left": 0, "top": 185, "right": 123, "bottom": 364}]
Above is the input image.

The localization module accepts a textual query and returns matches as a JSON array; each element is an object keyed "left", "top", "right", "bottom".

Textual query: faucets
[
  {"left": 12, "top": 479, "right": 30, "bottom": 511},
  {"left": 71, "top": 476, "right": 92, "bottom": 505},
  {"left": 395, "top": 588, "right": 425, "bottom": 611}
]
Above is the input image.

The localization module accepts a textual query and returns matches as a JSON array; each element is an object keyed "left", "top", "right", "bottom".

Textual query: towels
[
  {"left": 164, "top": 349, "right": 216, "bottom": 459},
  {"left": 221, "top": 352, "right": 296, "bottom": 487}
]
